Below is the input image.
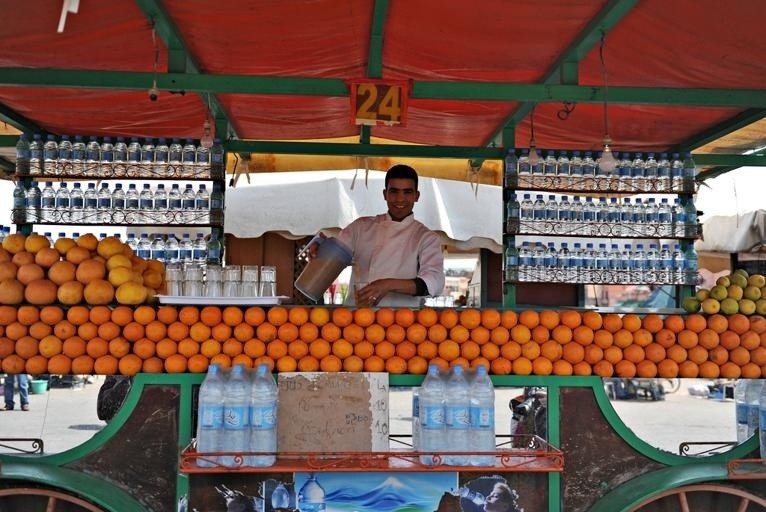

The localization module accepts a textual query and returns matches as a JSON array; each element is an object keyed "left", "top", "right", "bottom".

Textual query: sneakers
[
  {"left": 0, "top": 405, "right": 13, "bottom": 411},
  {"left": 22, "top": 404, "right": 29, "bottom": 411}
]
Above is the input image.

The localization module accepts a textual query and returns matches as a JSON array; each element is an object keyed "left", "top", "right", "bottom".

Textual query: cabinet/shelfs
[
  {"left": 18, "top": 132, "right": 223, "bottom": 266},
  {"left": 501, "top": 149, "right": 702, "bottom": 306}
]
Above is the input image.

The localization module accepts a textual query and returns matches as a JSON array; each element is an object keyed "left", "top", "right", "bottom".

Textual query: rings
[{"left": 370, "top": 294, "right": 377, "bottom": 301}]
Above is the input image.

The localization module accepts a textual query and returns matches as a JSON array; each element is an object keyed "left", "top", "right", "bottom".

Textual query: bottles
[
  {"left": 296, "top": 472, "right": 326, "bottom": 512},
  {"left": 735, "top": 379, "right": 765, "bottom": 467},
  {"left": 195, "top": 360, "right": 279, "bottom": 470},
  {"left": 502, "top": 147, "right": 699, "bottom": 284},
  {"left": 0, "top": 131, "right": 225, "bottom": 266},
  {"left": 409, "top": 363, "right": 498, "bottom": 468},
  {"left": 270, "top": 483, "right": 291, "bottom": 511},
  {"left": 459, "top": 486, "right": 486, "bottom": 506}
]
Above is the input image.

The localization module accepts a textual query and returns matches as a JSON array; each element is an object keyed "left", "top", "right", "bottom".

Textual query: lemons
[{"left": 684, "top": 268, "right": 766, "bottom": 314}]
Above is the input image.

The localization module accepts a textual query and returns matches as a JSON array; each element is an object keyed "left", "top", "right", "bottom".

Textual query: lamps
[
  {"left": 599, "top": 30, "right": 617, "bottom": 174},
  {"left": 200, "top": 92, "right": 214, "bottom": 149},
  {"left": 529, "top": 105, "right": 540, "bottom": 167}
]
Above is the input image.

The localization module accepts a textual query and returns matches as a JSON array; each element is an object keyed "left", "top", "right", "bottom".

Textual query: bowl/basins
[{"left": 31, "top": 380, "right": 48, "bottom": 393}]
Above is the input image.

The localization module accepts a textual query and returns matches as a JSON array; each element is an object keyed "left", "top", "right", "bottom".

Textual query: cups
[
  {"left": 354, "top": 281, "right": 370, "bottom": 307},
  {"left": 291, "top": 228, "right": 355, "bottom": 303},
  {"left": 164, "top": 261, "right": 278, "bottom": 300}
]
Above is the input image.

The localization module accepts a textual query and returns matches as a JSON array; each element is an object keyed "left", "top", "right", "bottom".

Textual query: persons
[
  {"left": 97, "top": 374, "right": 134, "bottom": 424},
  {"left": 309, "top": 164, "right": 445, "bottom": 306},
  {"left": 1, "top": 374, "right": 34, "bottom": 412}
]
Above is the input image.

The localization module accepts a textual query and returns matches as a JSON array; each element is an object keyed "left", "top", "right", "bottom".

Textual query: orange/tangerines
[{"left": 0, "top": 307, "right": 766, "bottom": 379}]
